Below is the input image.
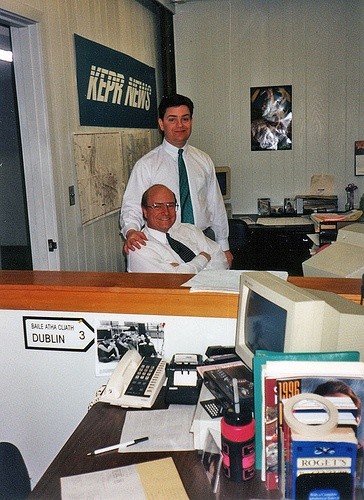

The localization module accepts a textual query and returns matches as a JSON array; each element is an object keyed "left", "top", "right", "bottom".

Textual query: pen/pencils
[{"left": 86, "top": 437, "right": 150, "bottom": 456}]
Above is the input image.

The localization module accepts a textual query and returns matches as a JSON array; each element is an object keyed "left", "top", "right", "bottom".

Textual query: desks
[
  {"left": 232, "top": 214, "right": 337, "bottom": 275},
  {"left": 289, "top": 428, "right": 358, "bottom": 500},
  {"left": 27, "top": 386, "right": 284, "bottom": 500}
]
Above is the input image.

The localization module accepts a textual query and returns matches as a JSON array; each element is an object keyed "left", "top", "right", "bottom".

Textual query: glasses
[{"left": 146, "top": 204, "right": 179, "bottom": 211}]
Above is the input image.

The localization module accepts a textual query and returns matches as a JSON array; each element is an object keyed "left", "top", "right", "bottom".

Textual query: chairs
[{"left": 204, "top": 219, "right": 277, "bottom": 272}]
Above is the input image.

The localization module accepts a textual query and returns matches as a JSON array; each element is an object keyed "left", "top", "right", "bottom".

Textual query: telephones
[{"left": 99, "top": 349, "right": 168, "bottom": 409}]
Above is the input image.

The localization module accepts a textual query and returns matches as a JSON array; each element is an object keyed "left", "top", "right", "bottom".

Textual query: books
[{"left": 264, "top": 377, "right": 364, "bottom": 500}]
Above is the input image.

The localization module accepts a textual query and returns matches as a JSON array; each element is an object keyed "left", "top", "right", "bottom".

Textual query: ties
[
  {"left": 165, "top": 232, "right": 196, "bottom": 263},
  {"left": 178, "top": 149, "right": 194, "bottom": 225}
]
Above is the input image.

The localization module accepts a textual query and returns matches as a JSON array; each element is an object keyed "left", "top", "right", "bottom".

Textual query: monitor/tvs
[
  {"left": 215, "top": 166, "right": 231, "bottom": 200},
  {"left": 235, "top": 272, "right": 364, "bottom": 372}
]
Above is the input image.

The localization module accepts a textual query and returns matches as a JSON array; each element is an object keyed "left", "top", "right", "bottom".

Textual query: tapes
[{"left": 283, "top": 393, "right": 339, "bottom": 436}]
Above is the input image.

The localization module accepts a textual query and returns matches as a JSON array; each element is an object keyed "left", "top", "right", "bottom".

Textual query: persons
[
  {"left": 97, "top": 333, "right": 157, "bottom": 363},
  {"left": 126, "top": 185, "right": 230, "bottom": 274},
  {"left": 120, "top": 94, "right": 234, "bottom": 268},
  {"left": 313, "top": 380, "right": 364, "bottom": 487}
]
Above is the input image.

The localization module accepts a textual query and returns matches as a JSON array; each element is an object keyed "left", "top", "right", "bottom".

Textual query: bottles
[{"left": 221, "top": 407, "right": 256, "bottom": 482}]
